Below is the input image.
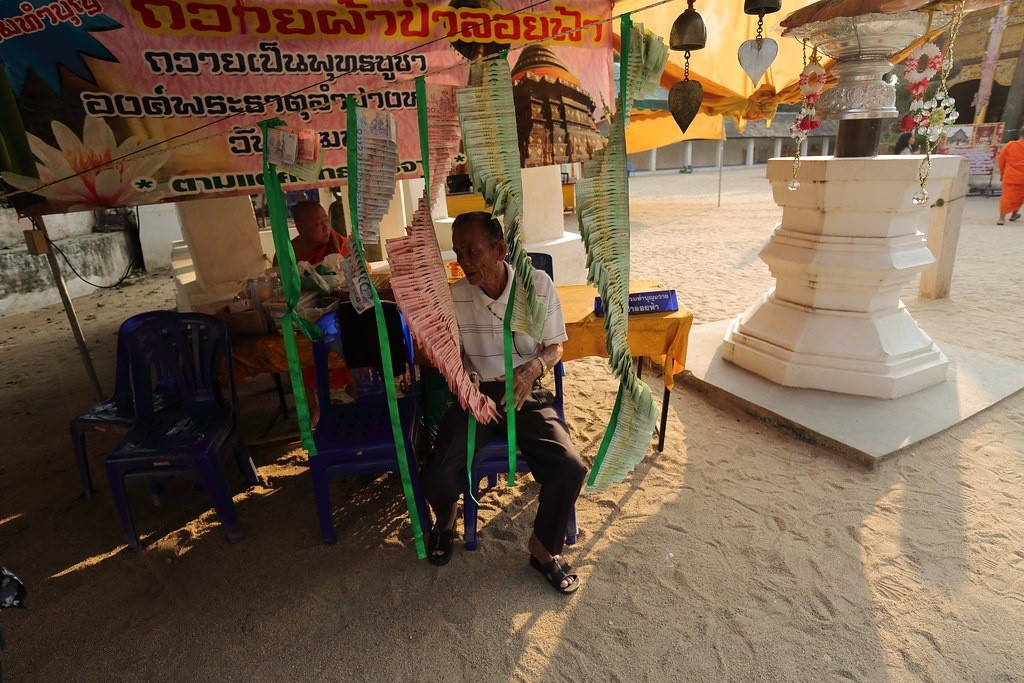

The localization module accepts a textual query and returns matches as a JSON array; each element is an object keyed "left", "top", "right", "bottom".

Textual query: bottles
[{"left": 243, "top": 273, "right": 284, "bottom": 303}]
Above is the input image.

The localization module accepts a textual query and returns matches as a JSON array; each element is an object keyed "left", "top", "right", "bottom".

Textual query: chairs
[
  {"left": 105, "top": 306, "right": 260, "bottom": 555},
  {"left": 460, "top": 252, "right": 575, "bottom": 552},
  {"left": 309, "top": 303, "right": 435, "bottom": 548},
  {"left": 70, "top": 309, "right": 175, "bottom": 502}
]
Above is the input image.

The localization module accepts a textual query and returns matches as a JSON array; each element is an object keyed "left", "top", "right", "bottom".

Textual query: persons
[
  {"left": 893, "top": 132, "right": 916, "bottom": 155},
  {"left": 273, "top": 200, "right": 371, "bottom": 430},
  {"left": 997, "top": 126, "right": 1024, "bottom": 225},
  {"left": 428, "top": 212, "right": 581, "bottom": 595}
]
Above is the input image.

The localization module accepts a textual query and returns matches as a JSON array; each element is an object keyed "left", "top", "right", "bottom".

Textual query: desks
[
  {"left": 149, "top": 333, "right": 354, "bottom": 446},
  {"left": 555, "top": 281, "right": 694, "bottom": 453}
]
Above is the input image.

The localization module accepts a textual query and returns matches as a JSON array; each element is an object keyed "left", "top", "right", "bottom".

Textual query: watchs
[{"left": 538, "top": 357, "right": 549, "bottom": 379}]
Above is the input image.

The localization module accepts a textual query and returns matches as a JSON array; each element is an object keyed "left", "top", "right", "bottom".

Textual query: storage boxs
[{"left": 216, "top": 291, "right": 340, "bottom": 338}]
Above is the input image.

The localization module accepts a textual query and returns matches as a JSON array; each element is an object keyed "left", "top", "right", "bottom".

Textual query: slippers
[
  {"left": 1009, "top": 214, "right": 1021, "bottom": 221},
  {"left": 426, "top": 517, "right": 457, "bottom": 566},
  {"left": 530, "top": 554, "right": 580, "bottom": 595},
  {"left": 998, "top": 222, "right": 1004, "bottom": 225}
]
